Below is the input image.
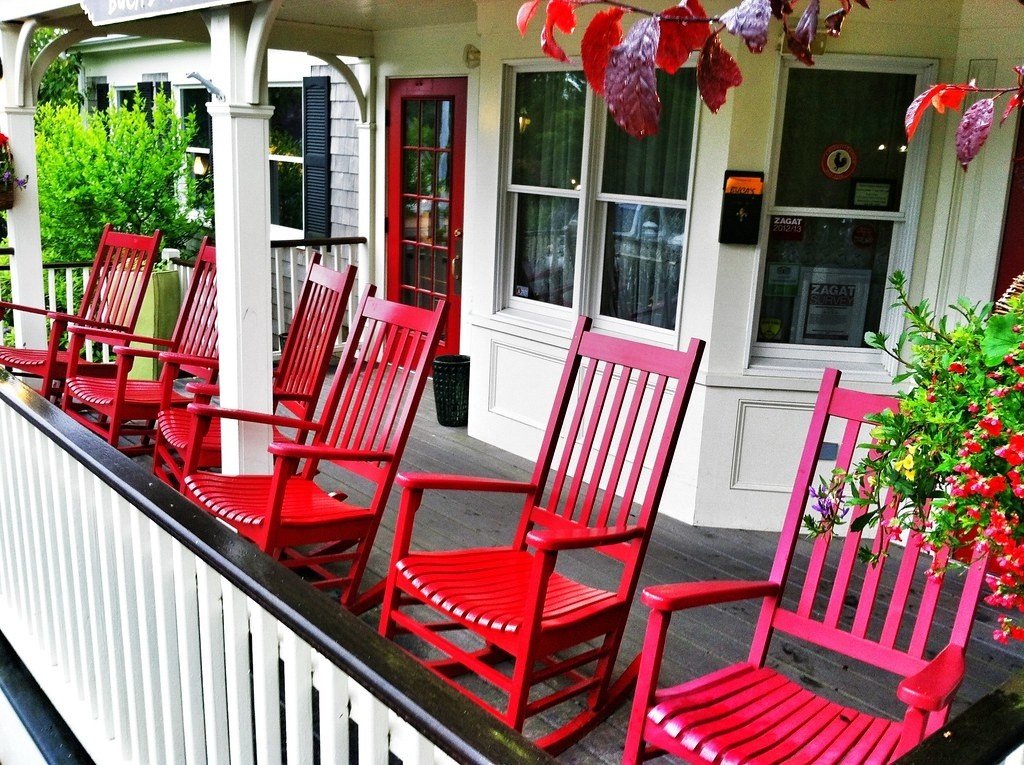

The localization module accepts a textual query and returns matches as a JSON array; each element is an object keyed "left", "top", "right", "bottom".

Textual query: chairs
[
  {"left": 0, "top": 222, "right": 163, "bottom": 403},
  {"left": 366, "top": 313, "right": 707, "bottom": 761},
  {"left": 181, "top": 282, "right": 451, "bottom": 609},
  {"left": 59, "top": 234, "right": 217, "bottom": 459},
  {"left": 616, "top": 366, "right": 994, "bottom": 765},
  {"left": 150, "top": 251, "right": 358, "bottom": 495}
]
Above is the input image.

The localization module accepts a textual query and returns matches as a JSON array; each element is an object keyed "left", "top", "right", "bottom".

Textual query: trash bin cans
[{"left": 431, "top": 355, "right": 470, "bottom": 427}]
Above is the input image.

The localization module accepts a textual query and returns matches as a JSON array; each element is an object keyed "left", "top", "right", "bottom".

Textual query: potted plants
[{"left": 21, "top": 84, "right": 220, "bottom": 384}]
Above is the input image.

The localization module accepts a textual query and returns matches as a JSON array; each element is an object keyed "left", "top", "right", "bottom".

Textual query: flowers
[
  {"left": 800, "top": 269, "right": 1023, "bottom": 652},
  {"left": 0, "top": 133, "right": 31, "bottom": 192}
]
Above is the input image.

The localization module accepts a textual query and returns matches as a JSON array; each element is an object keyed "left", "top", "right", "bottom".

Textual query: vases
[{"left": 0, "top": 179, "right": 16, "bottom": 211}]
